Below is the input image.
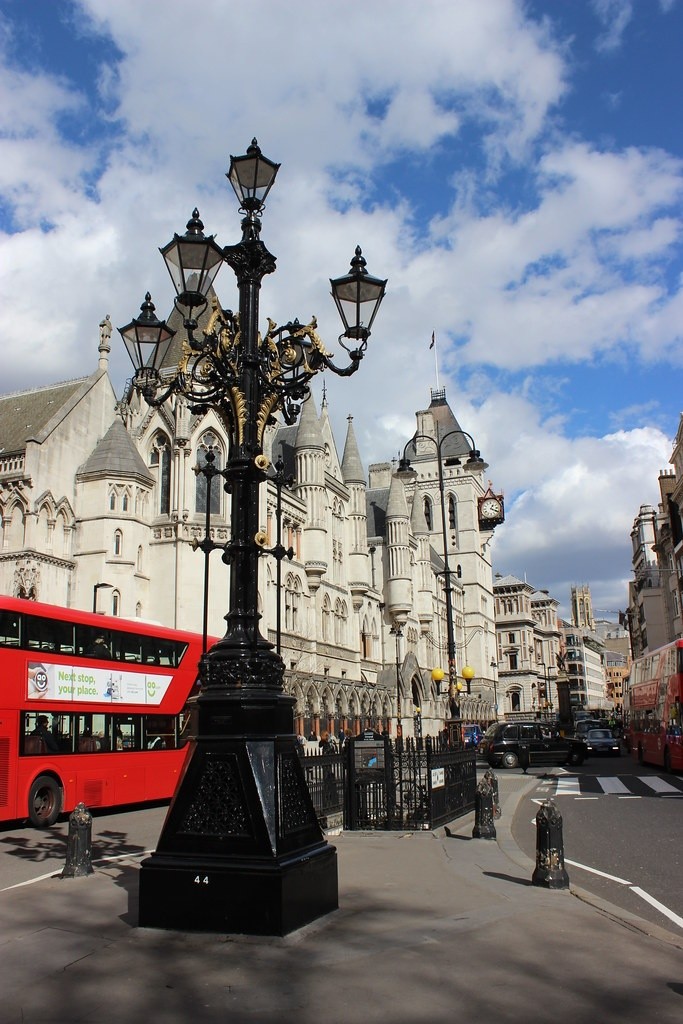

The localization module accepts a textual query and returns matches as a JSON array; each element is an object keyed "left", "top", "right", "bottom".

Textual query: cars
[{"left": 573, "top": 706, "right": 622, "bottom": 755}]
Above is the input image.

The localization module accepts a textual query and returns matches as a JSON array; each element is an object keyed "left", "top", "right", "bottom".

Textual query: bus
[
  {"left": 616, "top": 673, "right": 631, "bottom": 754},
  {"left": 0, "top": 593, "right": 223, "bottom": 832},
  {"left": 626, "top": 636, "right": 683, "bottom": 772}
]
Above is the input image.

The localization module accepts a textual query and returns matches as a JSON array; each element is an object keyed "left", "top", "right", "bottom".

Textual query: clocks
[{"left": 480, "top": 498, "right": 502, "bottom": 519}]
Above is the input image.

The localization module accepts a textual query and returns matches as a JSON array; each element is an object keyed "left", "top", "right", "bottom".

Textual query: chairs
[
  {"left": 24, "top": 735, "right": 47, "bottom": 755},
  {"left": 77, "top": 736, "right": 97, "bottom": 753},
  {"left": 0, "top": 640, "right": 157, "bottom": 666}
]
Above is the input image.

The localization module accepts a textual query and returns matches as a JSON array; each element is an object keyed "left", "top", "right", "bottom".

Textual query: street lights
[
  {"left": 538, "top": 662, "right": 548, "bottom": 718},
  {"left": 431, "top": 665, "right": 476, "bottom": 742},
  {"left": 92, "top": 583, "right": 114, "bottom": 613},
  {"left": 114, "top": 135, "right": 389, "bottom": 940},
  {"left": 547, "top": 666, "right": 556, "bottom": 719},
  {"left": 415, "top": 705, "right": 423, "bottom": 750},
  {"left": 389, "top": 624, "right": 405, "bottom": 751},
  {"left": 490, "top": 662, "right": 498, "bottom": 722},
  {"left": 391, "top": 419, "right": 491, "bottom": 744}
]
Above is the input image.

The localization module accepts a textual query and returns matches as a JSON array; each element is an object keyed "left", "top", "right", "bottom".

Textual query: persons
[
  {"left": 30, "top": 716, "right": 175, "bottom": 754},
  {"left": 609, "top": 716, "right": 623, "bottom": 737},
  {"left": 541, "top": 730, "right": 550, "bottom": 739},
  {"left": 83, "top": 634, "right": 112, "bottom": 659},
  {"left": 28, "top": 667, "right": 48, "bottom": 698},
  {"left": 555, "top": 651, "right": 568, "bottom": 672},
  {"left": 297, "top": 727, "right": 345, "bottom": 780}
]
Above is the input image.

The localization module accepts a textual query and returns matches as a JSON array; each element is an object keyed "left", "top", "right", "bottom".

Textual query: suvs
[
  {"left": 477, "top": 720, "right": 589, "bottom": 769},
  {"left": 464, "top": 724, "right": 485, "bottom": 748}
]
